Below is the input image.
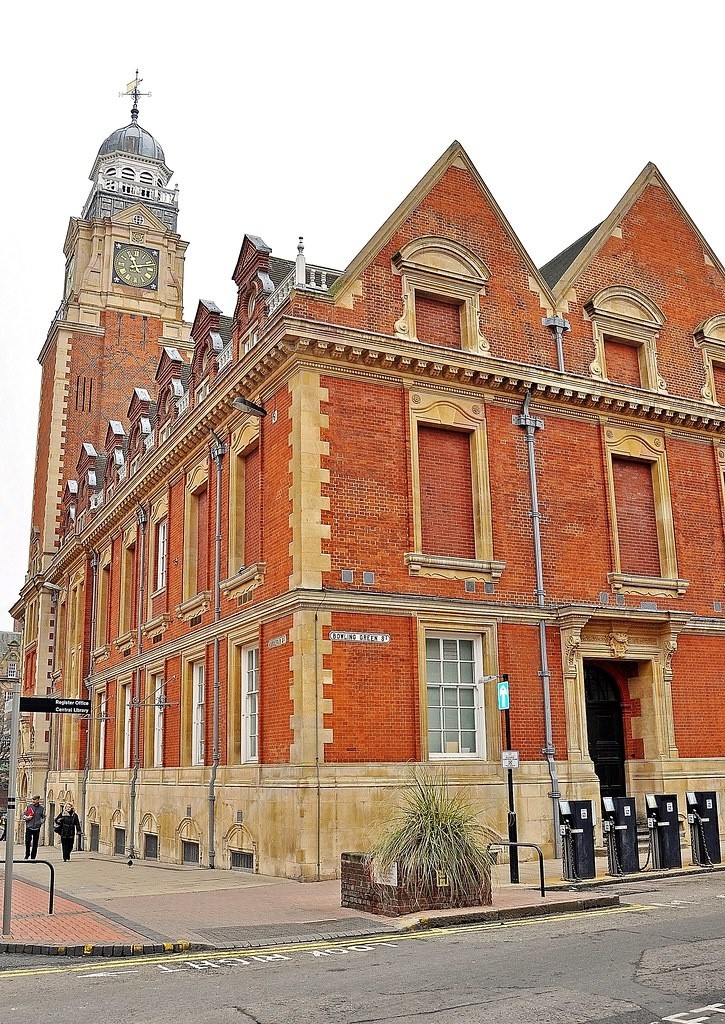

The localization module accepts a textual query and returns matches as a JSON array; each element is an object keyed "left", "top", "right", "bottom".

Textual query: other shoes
[
  {"left": 63, "top": 859, "right": 70, "bottom": 862},
  {"left": 24, "top": 854, "right": 30, "bottom": 859}
]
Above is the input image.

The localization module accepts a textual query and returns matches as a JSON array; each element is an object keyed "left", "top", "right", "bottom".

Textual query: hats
[{"left": 32, "top": 795, "right": 40, "bottom": 800}]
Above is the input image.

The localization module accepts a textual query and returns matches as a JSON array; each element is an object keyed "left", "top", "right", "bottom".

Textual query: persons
[
  {"left": 0, "top": 814, "right": 7, "bottom": 841},
  {"left": 22, "top": 795, "right": 46, "bottom": 859},
  {"left": 54, "top": 802, "right": 81, "bottom": 862}
]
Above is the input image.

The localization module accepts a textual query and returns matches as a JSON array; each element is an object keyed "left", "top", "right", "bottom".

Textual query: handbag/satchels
[{"left": 53, "top": 821, "right": 64, "bottom": 835}]
